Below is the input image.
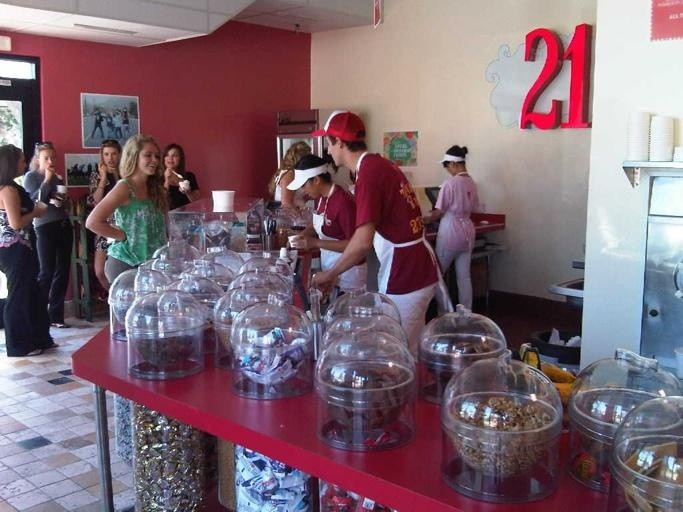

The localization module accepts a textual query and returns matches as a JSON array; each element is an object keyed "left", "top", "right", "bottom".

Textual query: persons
[
  {"left": 86, "top": 140, "right": 123, "bottom": 292},
  {"left": 422, "top": 144, "right": 478, "bottom": 318},
  {"left": 162, "top": 143, "right": 201, "bottom": 211},
  {"left": 309, "top": 110, "right": 438, "bottom": 360},
  {"left": 1, "top": 145, "right": 59, "bottom": 357},
  {"left": 89, "top": 106, "right": 130, "bottom": 139},
  {"left": 23, "top": 141, "right": 75, "bottom": 328},
  {"left": 270, "top": 142, "right": 312, "bottom": 210},
  {"left": 287, "top": 155, "right": 368, "bottom": 298},
  {"left": 86, "top": 134, "right": 171, "bottom": 284}
]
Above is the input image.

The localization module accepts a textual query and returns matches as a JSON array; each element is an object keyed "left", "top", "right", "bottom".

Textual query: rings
[{"left": 312, "top": 272, "right": 317, "bottom": 278}]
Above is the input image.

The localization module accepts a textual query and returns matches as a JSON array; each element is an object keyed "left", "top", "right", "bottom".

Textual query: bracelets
[
  {"left": 98, "top": 179, "right": 106, "bottom": 183},
  {"left": 97, "top": 187, "right": 105, "bottom": 192}
]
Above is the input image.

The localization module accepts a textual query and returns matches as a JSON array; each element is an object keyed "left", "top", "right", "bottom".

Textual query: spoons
[
  {"left": 171, "top": 170, "right": 182, "bottom": 179},
  {"left": 54, "top": 172, "right": 62, "bottom": 180}
]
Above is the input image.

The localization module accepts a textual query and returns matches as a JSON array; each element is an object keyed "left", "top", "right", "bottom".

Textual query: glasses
[{"left": 35, "top": 141, "right": 53, "bottom": 148}]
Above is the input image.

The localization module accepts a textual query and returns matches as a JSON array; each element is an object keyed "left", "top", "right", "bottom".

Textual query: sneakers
[
  {"left": 24, "top": 349, "right": 43, "bottom": 355},
  {"left": 50, "top": 321, "right": 67, "bottom": 328},
  {"left": 43, "top": 342, "right": 60, "bottom": 349}
]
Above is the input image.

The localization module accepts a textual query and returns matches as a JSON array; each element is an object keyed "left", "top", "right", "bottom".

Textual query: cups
[
  {"left": 179, "top": 182, "right": 188, "bottom": 191},
  {"left": 279, "top": 247, "right": 288, "bottom": 258},
  {"left": 628, "top": 105, "right": 683, "bottom": 163},
  {"left": 287, "top": 235, "right": 297, "bottom": 249},
  {"left": 56, "top": 185, "right": 66, "bottom": 193},
  {"left": 34, "top": 202, "right": 47, "bottom": 218},
  {"left": 261, "top": 234, "right": 275, "bottom": 251},
  {"left": 211, "top": 189, "right": 234, "bottom": 213}
]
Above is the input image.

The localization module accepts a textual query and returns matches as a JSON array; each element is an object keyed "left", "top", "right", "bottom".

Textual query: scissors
[{"left": 266, "top": 215, "right": 276, "bottom": 235}]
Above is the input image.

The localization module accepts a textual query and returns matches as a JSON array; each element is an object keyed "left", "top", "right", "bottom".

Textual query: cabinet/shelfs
[{"left": 65, "top": 194, "right": 110, "bottom": 324}]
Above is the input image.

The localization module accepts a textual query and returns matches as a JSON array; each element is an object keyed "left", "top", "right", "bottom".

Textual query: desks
[
  {"left": 425, "top": 209, "right": 509, "bottom": 249},
  {"left": 67, "top": 242, "right": 641, "bottom": 512}
]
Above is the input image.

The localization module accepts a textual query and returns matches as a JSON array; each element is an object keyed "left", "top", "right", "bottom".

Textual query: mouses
[{"left": 480, "top": 220, "right": 490, "bottom": 225}]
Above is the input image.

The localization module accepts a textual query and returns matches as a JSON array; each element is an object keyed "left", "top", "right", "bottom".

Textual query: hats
[
  {"left": 286, "top": 162, "right": 328, "bottom": 191},
  {"left": 311, "top": 110, "right": 366, "bottom": 142},
  {"left": 439, "top": 153, "right": 465, "bottom": 164}
]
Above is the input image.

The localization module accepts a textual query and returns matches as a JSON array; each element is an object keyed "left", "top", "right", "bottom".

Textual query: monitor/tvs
[{"left": 424, "top": 187, "right": 441, "bottom": 211}]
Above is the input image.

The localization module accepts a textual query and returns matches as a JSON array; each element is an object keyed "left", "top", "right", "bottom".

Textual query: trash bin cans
[{"left": 529, "top": 329, "right": 582, "bottom": 376}]
